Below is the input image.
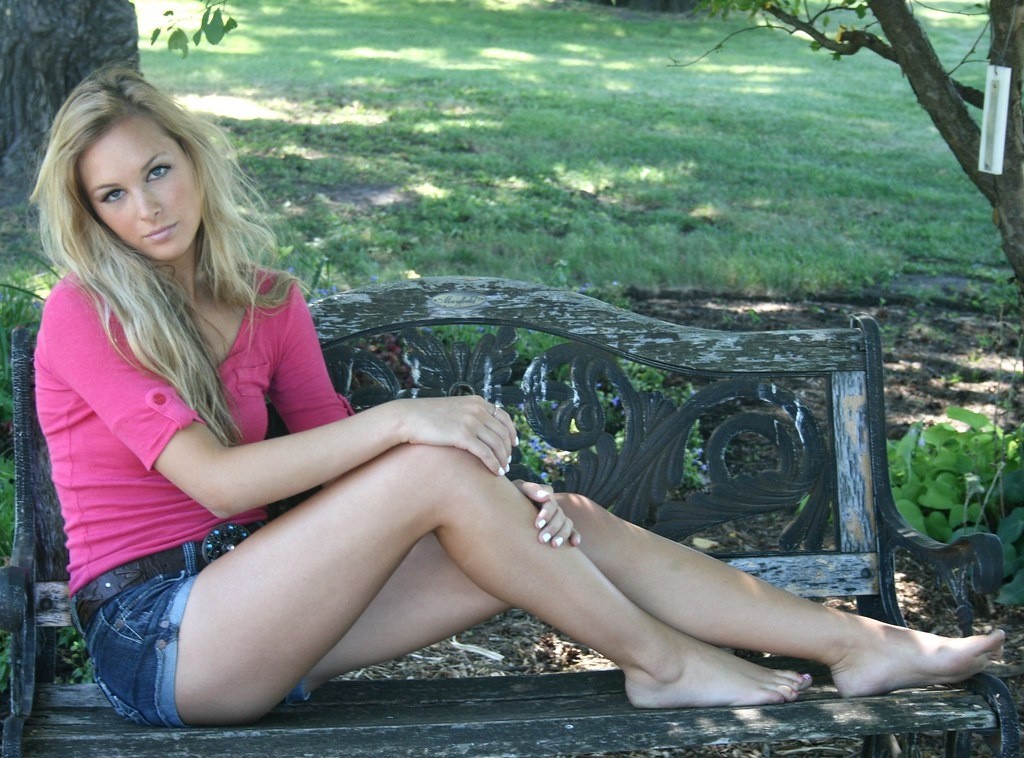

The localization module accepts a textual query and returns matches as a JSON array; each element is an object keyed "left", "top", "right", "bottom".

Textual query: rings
[{"left": 494, "top": 405, "right": 501, "bottom": 418}]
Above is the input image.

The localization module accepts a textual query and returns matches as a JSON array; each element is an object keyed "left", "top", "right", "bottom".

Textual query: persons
[{"left": 28, "top": 65, "right": 1006, "bottom": 731}]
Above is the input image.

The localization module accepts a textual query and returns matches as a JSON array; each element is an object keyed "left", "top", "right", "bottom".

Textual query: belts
[{"left": 72, "top": 520, "right": 266, "bottom": 633}]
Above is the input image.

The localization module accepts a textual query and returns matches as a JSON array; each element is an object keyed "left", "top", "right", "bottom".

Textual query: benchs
[{"left": 1, "top": 272, "right": 1023, "bottom": 758}]
